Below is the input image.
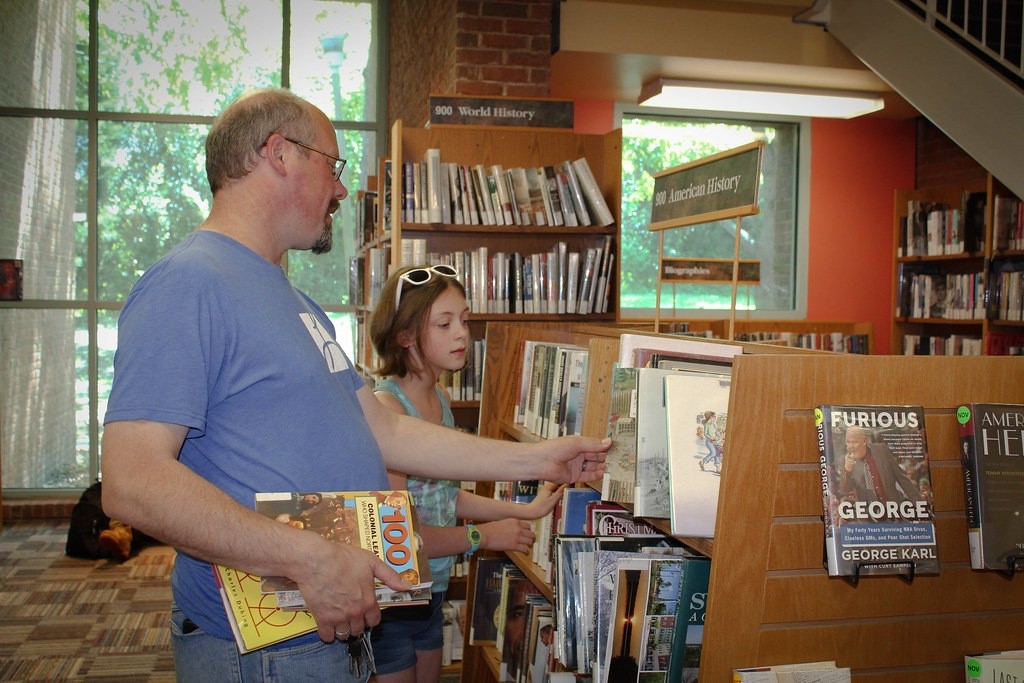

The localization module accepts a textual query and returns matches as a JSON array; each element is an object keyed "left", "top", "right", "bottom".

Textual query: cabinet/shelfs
[{"left": 355, "top": 119, "right": 1024, "bottom": 683}]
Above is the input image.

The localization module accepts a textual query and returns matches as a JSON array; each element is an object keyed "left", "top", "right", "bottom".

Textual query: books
[
  {"left": 209, "top": 489, "right": 434, "bottom": 657},
  {"left": 355, "top": 148, "right": 735, "bottom": 683},
  {"left": 955, "top": 403, "right": 1024, "bottom": 573},
  {"left": 268, "top": 492, "right": 347, "bottom": 532},
  {"left": 963, "top": 647, "right": 1024, "bottom": 683},
  {"left": 813, "top": 404, "right": 942, "bottom": 580},
  {"left": 738, "top": 330, "right": 872, "bottom": 354},
  {"left": 893, "top": 188, "right": 1024, "bottom": 357},
  {"left": 732, "top": 660, "right": 853, "bottom": 683}
]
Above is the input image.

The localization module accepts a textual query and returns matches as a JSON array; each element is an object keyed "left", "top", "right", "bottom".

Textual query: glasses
[
  {"left": 396, "top": 264, "right": 458, "bottom": 312},
  {"left": 263, "top": 137, "right": 347, "bottom": 181}
]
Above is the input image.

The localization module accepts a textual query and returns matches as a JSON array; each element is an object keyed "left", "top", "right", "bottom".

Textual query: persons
[
  {"left": 830, "top": 426, "right": 935, "bottom": 523},
  {"left": 504, "top": 579, "right": 540, "bottom": 678},
  {"left": 96, "top": 89, "right": 612, "bottom": 683},
  {"left": 370, "top": 264, "right": 572, "bottom": 683},
  {"left": 538, "top": 623, "right": 553, "bottom": 646}
]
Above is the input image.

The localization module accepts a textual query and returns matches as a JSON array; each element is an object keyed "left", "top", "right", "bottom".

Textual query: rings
[
  {"left": 335, "top": 627, "right": 351, "bottom": 640},
  {"left": 582, "top": 460, "right": 589, "bottom": 471}
]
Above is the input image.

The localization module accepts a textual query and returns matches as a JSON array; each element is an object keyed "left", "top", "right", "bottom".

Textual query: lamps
[{"left": 637, "top": 78, "right": 885, "bottom": 119}]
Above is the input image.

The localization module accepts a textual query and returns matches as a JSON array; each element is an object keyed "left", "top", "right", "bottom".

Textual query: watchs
[{"left": 465, "top": 522, "right": 481, "bottom": 557}]
